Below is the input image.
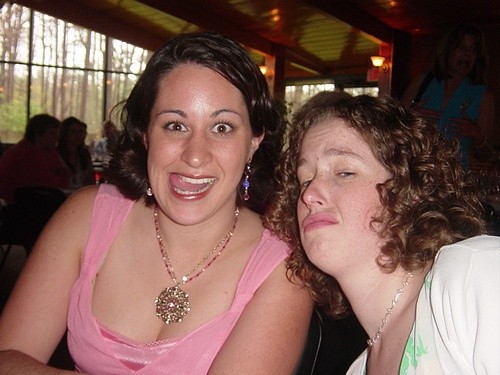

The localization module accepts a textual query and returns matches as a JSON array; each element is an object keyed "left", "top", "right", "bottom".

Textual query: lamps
[{"left": 371, "top": 56, "right": 390, "bottom": 74}]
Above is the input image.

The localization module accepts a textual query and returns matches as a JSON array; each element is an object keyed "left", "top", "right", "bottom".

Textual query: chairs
[{"left": 9, "top": 186, "right": 67, "bottom": 260}]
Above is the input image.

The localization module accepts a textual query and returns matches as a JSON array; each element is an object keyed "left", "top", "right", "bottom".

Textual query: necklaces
[
  {"left": 366, "top": 272, "right": 413, "bottom": 347},
  {"left": 153, "top": 204, "right": 240, "bottom": 323}
]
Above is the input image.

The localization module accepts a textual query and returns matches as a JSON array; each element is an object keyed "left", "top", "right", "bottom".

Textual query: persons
[
  {"left": 261, "top": 92, "right": 499, "bottom": 375},
  {"left": 1, "top": 115, "right": 119, "bottom": 210},
  {"left": 1, "top": 32, "right": 314, "bottom": 375}
]
